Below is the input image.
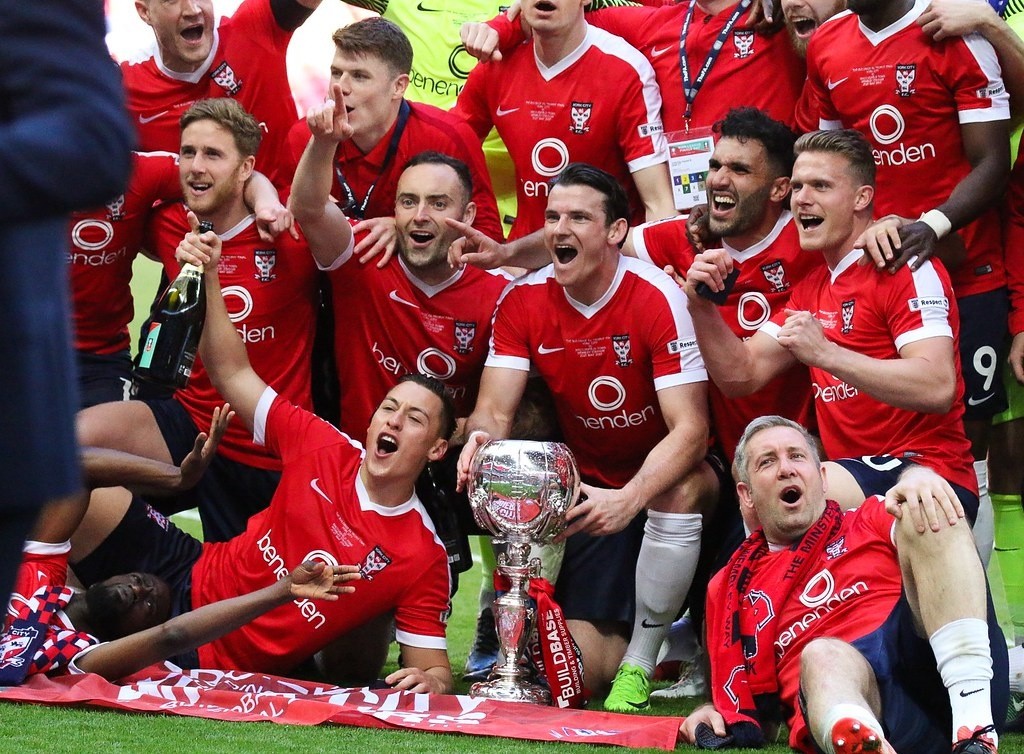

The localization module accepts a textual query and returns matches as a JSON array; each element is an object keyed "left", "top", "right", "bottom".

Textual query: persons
[
  {"left": 0, "top": 0, "right": 1024, "bottom": 733},
  {"left": 69, "top": 214, "right": 458, "bottom": 695},
  {"left": 455, "top": 163, "right": 709, "bottom": 689},
  {"left": 79, "top": 100, "right": 314, "bottom": 540},
  {"left": 280, "top": 19, "right": 503, "bottom": 246},
  {"left": 677, "top": 416, "right": 1009, "bottom": 754}
]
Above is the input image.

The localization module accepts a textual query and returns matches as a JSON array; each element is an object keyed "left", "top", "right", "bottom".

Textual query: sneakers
[
  {"left": 461, "top": 607, "right": 498, "bottom": 681},
  {"left": 603, "top": 662, "right": 655, "bottom": 714}
]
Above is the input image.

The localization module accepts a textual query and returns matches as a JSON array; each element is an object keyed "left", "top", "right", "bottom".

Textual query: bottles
[{"left": 132, "top": 221, "right": 214, "bottom": 394}]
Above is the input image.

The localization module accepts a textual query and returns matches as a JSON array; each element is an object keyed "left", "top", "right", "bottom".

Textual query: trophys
[{"left": 464, "top": 439, "right": 582, "bottom": 703}]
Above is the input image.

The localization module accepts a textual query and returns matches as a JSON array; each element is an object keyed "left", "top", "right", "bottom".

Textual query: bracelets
[{"left": 917, "top": 210, "right": 950, "bottom": 241}]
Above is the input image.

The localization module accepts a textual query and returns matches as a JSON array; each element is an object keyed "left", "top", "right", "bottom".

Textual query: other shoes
[
  {"left": 823, "top": 715, "right": 898, "bottom": 754},
  {"left": 951, "top": 724, "right": 998, "bottom": 754}
]
